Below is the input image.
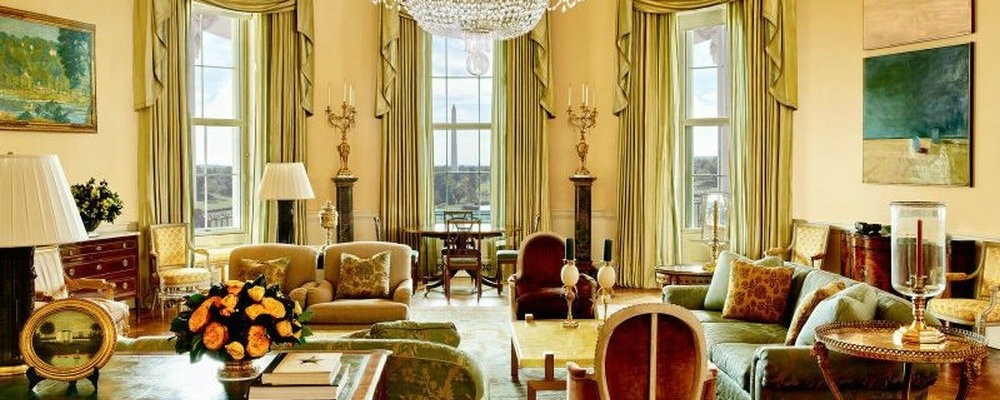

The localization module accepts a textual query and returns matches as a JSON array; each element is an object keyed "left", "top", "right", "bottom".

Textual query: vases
[
  {"left": 83, "top": 218, "right": 102, "bottom": 232},
  {"left": 217, "top": 355, "right": 262, "bottom": 399}
]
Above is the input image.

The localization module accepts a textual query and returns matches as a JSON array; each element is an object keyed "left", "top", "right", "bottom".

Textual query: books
[{"left": 249, "top": 351, "right": 351, "bottom": 400}]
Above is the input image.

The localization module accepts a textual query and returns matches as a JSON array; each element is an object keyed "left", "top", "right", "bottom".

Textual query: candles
[
  {"left": 714, "top": 200, "right": 720, "bottom": 237},
  {"left": 567, "top": 80, "right": 599, "bottom": 110},
  {"left": 326, "top": 82, "right": 359, "bottom": 113},
  {"left": 916, "top": 216, "right": 923, "bottom": 275}
]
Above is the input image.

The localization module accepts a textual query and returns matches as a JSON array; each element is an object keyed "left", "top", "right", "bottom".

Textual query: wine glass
[
  {"left": 890, "top": 202, "right": 949, "bottom": 343},
  {"left": 701, "top": 192, "right": 730, "bottom": 270}
]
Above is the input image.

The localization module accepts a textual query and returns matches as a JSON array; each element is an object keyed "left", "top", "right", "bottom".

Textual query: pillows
[
  {"left": 238, "top": 255, "right": 289, "bottom": 299},
  {"left": 707, "top": 251, "right": 881, "bottom": 346},
  {"left": 337, "top": 250, "right": 392, "bottom": 300},
  {"left": 363, "top": 322, "right": 462, "bottom": 349}
]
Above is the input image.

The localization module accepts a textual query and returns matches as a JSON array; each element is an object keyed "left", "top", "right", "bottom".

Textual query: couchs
[
  {"left": 229, "top": 242, "right": 414, "bottom": 334},
  {"left": 663, "top": 256, "right": 943, "bottom": 400},
  {"left": 121, "top": 322, "right": 487, "bottom": 399}
]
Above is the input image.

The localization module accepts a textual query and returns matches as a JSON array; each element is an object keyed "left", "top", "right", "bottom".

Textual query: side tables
[{"left": 810, "top": 327, "right": 987, "bottom": 400}]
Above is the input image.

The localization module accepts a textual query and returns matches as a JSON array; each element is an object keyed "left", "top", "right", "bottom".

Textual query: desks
[
  {"left": 402, "top": 226, "right": 521, "bottom": 299},
  {"left": 62, "top": 230, "right": 142, "bottom": 329},
  {"left": 0, "top": 349, "right": 396, "bottom": 400},
  {"left": 653, "top": 264, "right": 716, "bottom": 290}
]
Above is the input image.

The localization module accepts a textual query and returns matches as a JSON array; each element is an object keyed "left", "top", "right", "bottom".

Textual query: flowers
[
  {"left": 172, "top": 278, "right": 311, "bottom": 372},
  {"left": 71, "top": 178, "right": 123, "bottom": 224}
]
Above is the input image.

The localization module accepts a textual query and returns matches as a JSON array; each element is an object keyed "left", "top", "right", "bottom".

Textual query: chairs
[
  {"left": 150, "top": 224, "right": 215, "bottom": 318},
  {"left": 508, "top": 231, "right": 599, "bottom": 323},
  {"left": 374, "top": 212, "right": 543, "bottom": 299},
  {"left": 567, "top": 301, "right": 706, "bottom": 400},
  {"left": 927, "top": 242, "right": 1000, "bottom": 342},
  {"left": 763, "top": 221, "right": 830, "bottom": 270}
]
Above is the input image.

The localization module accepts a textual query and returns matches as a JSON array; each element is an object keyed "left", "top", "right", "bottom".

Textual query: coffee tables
[{"left": 509, "top": 315, "right": 608, "bottom": 400}]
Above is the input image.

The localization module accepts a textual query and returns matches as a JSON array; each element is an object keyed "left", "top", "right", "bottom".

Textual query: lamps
[
  {"left": 0, "top": 155, "right": 90, "bottom": 366},
  {"left": 258, "top": 163, "right": 314, "bottom": 244},
  {"left": 372, "top": 0, "right": 581, "bottom": 76}
]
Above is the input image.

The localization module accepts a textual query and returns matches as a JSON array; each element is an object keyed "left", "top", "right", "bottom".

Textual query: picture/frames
[
  {"left": 0, "top": 8, "right": 98, "bottom": 134},
  {"left": 20, "top": 302, "right": 119, "bottom": 381}
]
